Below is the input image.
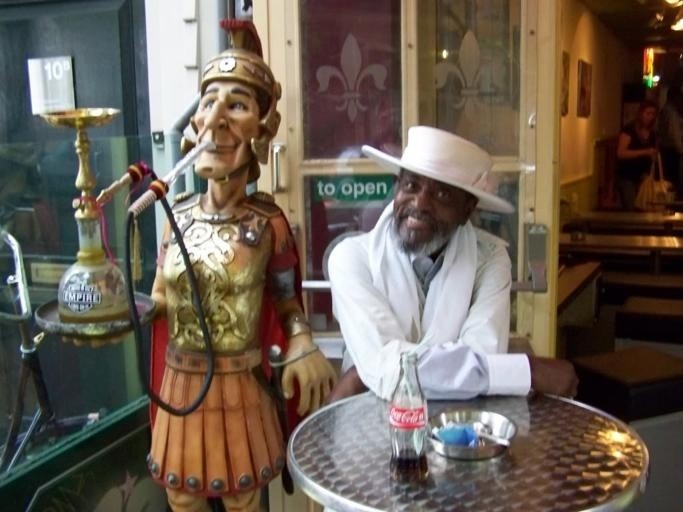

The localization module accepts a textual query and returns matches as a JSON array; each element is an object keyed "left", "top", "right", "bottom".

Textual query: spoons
[{"left": 472, "top": 421, "right": 512, "bottom": 447}]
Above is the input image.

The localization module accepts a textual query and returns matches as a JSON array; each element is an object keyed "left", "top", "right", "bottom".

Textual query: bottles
[{"left": 388, "top": 350, "right": 431, "bottom": 482}]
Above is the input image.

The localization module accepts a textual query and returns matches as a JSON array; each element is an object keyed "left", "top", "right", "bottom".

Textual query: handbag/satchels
[{"left": 635, "top": 153, "right": 672, "bottom": 213}]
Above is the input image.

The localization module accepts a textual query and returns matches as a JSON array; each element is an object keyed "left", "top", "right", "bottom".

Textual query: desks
[
  {"left": 286, "top": 389, "right": 650, "bottom": 512},
  {"left": 558, "top": 230, "right": 683, "bottom": 275},
  {"left": 573, "top": 211, "right": 683, "bottom": 234}
]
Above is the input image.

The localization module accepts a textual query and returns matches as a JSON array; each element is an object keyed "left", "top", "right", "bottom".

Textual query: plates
[{"left": 425, "top": 409, "right": 518, "bottom": 462}]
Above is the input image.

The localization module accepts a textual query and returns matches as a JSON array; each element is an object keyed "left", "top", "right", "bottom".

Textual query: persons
[
  {"left": 117, "top": 16, "right": 334, "bottom": 511},
  {"left": 326, "top": 127, "right": 584, "bottom": 408},
  {"left": 616, "top": 102, "right": 665, "bottom": 208},
  {"left": 662, "top": 81, "right": 682, "bottom": 193}
]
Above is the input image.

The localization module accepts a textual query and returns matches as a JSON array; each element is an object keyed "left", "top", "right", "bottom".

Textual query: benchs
[{"left": 568, "top": 271, "right": 683, "bottom": 421}]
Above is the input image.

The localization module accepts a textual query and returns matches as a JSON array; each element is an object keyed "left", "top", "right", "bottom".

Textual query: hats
[{"left": 362, "top": 126, "right": 515, "bottom": 214}]
